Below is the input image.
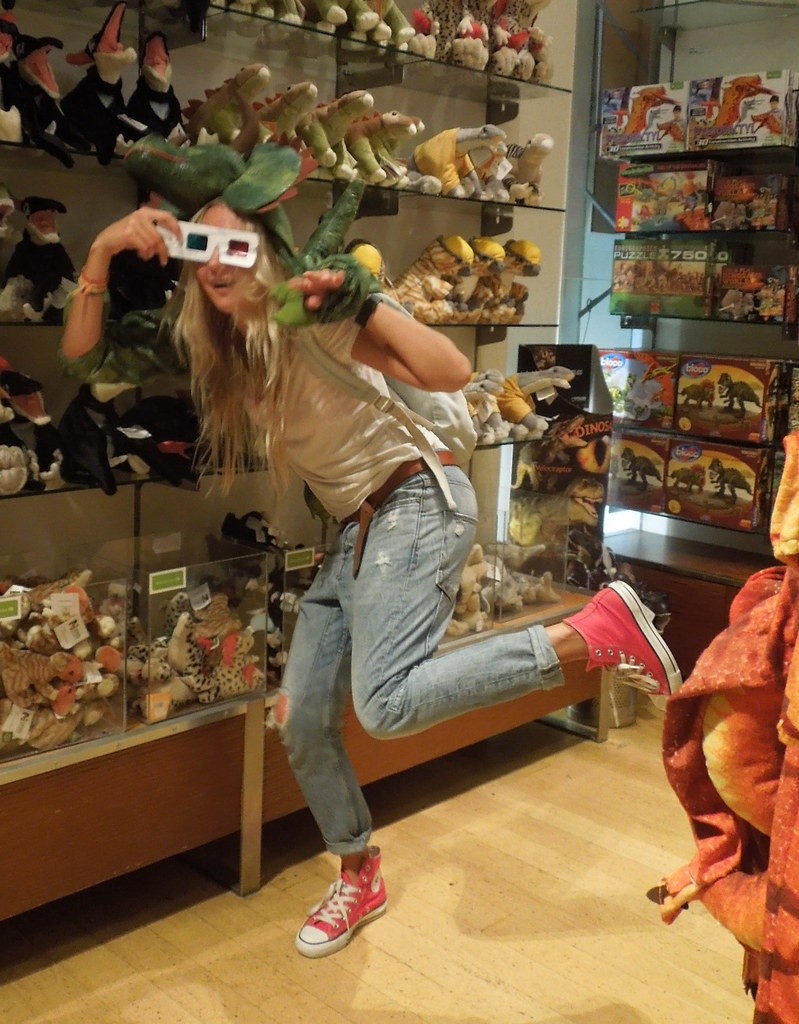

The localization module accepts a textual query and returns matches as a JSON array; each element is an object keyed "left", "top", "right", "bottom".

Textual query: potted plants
[{"left": 566, "top": 530, "right": 670, "bottom": 728}]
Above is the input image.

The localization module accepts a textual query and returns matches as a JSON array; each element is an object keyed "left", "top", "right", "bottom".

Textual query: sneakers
[
  {"left": 562, "top": 579, "right": 684, "bottom": 697},
  {"left": 294, "top": 845, "right": 388, "bottom": 958}
]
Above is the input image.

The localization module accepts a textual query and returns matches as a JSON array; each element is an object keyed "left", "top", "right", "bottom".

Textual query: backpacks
[{"left": 297, "top": 293, "right": 480, "bottom": 513}]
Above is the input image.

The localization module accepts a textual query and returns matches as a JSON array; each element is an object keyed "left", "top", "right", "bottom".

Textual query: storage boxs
[
  {"left": 616, "top": 154, "right": 714, "bottom": 231},
  {"left": 609, "top": 238, "right": 711, "bottom": 317},
  {"left": 597, "top": 348, "right": 681, "bottom": 433},
  {"left": 688, "top": 70, "right": 795, "bottom": 150},
  {"left": 491, "top": 487, "right": 569, "bottom": 627},
  {"left": 715, "top": 262, "right": 791, "bottom": 322},
  {"left": 83, "top": 531, "right": 269, "bottom": 725},
  {"left": 609, "top": 424, "right": 669, "bottom": 514},
  {"left": 672, "top": 355, "right": 775, "bottom": 446},
  {"left": 0, "top": 543, "right": 128, "bottom": 764},
  {"left": 221, "top": 510, "right": 335, "bottom": 690},
  {"left": 707, "top": 176, "right": 786, "bottom": 230},
  {"left": 666, "top": 436, "right": 769, "bottom": 533},
  {"left": 439, "top": 504, "right": 493, "bottom": 643},
  {"left": 600, "top": 77, "right": 685, "bottom": 158}
]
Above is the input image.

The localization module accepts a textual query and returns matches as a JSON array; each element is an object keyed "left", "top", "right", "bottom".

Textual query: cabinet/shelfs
[
  {"left": 606, "top": 136, "right": 796, "bottom": 535},
  {"left": 0, "top": 1, "right": 572, "bottom": 500}
]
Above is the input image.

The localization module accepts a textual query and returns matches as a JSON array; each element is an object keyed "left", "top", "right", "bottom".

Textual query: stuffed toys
[{"left": 2, "top": 1, "right": 579, "bottom": 750}]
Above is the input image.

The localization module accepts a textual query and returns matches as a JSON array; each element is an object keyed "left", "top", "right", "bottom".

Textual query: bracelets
[
  {"left": 356, "top": 295, "right": 383, "bottom": 327},
  {"left": 65, "top": 267, "right": 110, "bottom": 300}
]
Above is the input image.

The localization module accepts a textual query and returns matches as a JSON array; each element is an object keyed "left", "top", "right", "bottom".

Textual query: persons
[{"left": 55, "top": 194, "right": 682, "bottom": 960}]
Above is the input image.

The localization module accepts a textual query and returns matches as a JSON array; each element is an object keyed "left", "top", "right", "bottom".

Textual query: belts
[{"left": 339, "top": 450, "right": 462, "bottom": 580}]
